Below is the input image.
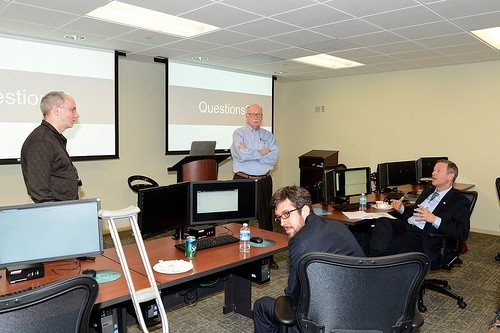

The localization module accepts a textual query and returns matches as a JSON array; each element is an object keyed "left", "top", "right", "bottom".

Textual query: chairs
[
  {"left": 275, "top": 251, "right": 432, "bottom": 333},
  {"left": 417, "top": 189, "right": 479, "bottom": 312},
  {"left": 0, "top": 275, "right": 99, "bottom": 333}
]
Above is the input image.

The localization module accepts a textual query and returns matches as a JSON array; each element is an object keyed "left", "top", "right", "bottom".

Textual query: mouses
[
  {"left": 407, "top": 192, "right": 416, "bottom": 196},
  {"left": 81, "top": 268, "right": 97, "bottom": 278},
  {"left": 251, "top": 236, "right": 262, "bottom": 244}
]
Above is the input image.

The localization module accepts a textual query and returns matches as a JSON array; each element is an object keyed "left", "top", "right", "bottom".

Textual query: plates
[
  {"left": 370, "top": 205, "right": 393, "bottom": 210},
  {"left": 153, "top": 260, "right": 193, "bottom": 274}
]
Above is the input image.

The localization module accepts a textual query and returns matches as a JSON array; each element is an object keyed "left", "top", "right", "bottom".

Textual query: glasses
[
  {"left": 57, "top": 107, "right": 76, "bottom": 113},
  {"left": 247, "top": 113, "right": 264, "bottom": 117},
  {"left": 274, "top": 206, "right": 302, "bottom": 224}
]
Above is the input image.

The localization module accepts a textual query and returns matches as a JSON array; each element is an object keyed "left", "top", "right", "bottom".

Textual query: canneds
[{"left": 185, "top": 236, "right": 197, "bottom": 258}]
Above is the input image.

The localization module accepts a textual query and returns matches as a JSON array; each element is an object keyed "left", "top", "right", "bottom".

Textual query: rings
[{"left": 421, "top": 214, "right": 423, "bottom": 218}]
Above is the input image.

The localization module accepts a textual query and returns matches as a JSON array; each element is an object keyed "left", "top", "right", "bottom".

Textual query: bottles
[
  {"left": 239, "top": 223, "right": 250, "bottom": 253},
  {"left": 359, "top": 193, "right": 367, "bottom": 212}
]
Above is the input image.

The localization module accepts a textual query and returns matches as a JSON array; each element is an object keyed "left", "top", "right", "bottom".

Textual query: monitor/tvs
[
  {"left": 190, "top": 179, "right": 259, "bottom": 225},
  {"left": 338, "top": 167, "right": 371, "bottom": 198},
  {"left": 376, "top": 163, "right": 388, "bottom": 191},
  {"left": 388, "top": 161, "right": 417, "bottom": 186},
  {"left": 137, "top": 181, "right": 191, "bottom": 239},
  {"left": 417, "top": 157, "right": 448, "bottom": 179},
  {"left": 323, "top": 168, "right": 336, "bottom": 206},
  {"left": 1, "top": 198, "right": 104, "bottom": 268}
]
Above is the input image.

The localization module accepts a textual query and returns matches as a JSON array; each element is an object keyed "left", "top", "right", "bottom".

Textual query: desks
[
  {"left": 303, "top": 180, "right": 476, "bottom": 227},
  {"left": 101, "top": 222, "right": 290, "bottom": 319},
  {"left": 0, "top": 254, "right": 160, "bottom": 333}
]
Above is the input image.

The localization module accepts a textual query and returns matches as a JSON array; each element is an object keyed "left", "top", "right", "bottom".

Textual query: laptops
[{"left": 189, "top": 141, "right": 217, "bottom": 155}]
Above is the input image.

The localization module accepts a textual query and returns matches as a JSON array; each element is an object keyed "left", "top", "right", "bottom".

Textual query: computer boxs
[
  {"left": 126, "top": 299, "right": 160, "bottom": 328},
  {"left": 226, "top": 256, "right": 273, "bottom": 285},
  {"left": 90, "top": 307, "right": 115, "bottom": 333}
]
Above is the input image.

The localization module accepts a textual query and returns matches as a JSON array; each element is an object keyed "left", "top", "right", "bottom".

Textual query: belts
[{"left": 236, "top": 172, "right": 270, "bottom": 181}]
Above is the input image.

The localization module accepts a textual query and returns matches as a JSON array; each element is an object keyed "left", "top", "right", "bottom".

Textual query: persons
[
  {"left": 21, "top": 91, "right": 80, "bottom": 203},
  {"left": 369, "top": 160, "right": 470, "bottom": 258},
  {"left": 230, "top": 103, "right": 280, "bottom": 231},
  {"left": 254, "top": 184, "right": 366, "bottom": 333}
]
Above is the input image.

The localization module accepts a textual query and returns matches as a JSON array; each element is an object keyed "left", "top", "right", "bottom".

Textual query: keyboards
[
  {"left": 334, "top": 201, "right": 376, "bottom": 211},
  {"left": 175, "top": 234, "right": 239, "bottom": 252},
  {"left": 386, "top": 193, "right": 405, "bottom": 201},
  {"left": 417, "top": 181, "right": 432, "bottom": 186}
]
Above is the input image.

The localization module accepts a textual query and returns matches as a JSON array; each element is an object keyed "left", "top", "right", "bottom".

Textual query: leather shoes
[{"left": 270, "top": 261, "right": 279, "bottom": 270}]
